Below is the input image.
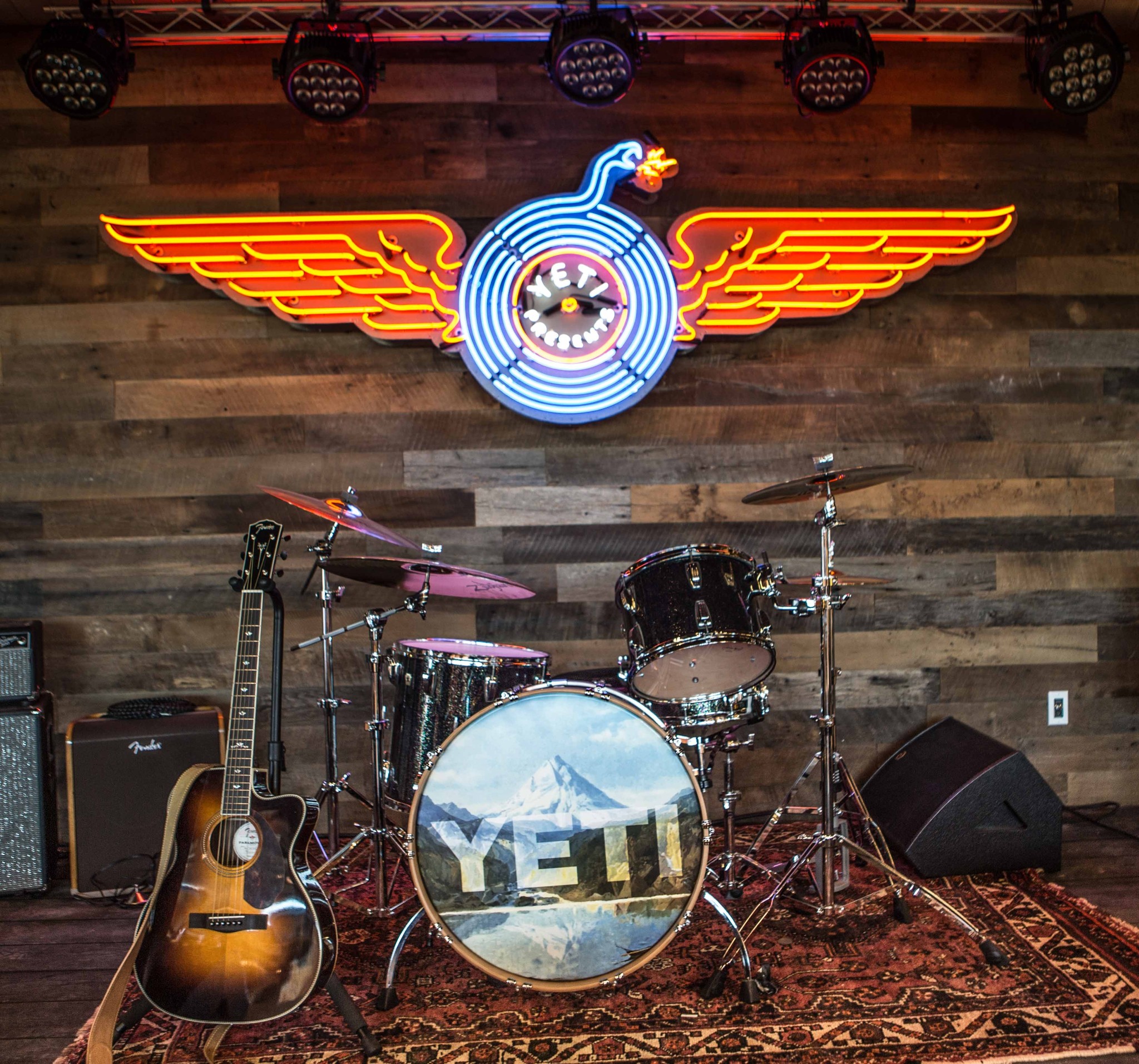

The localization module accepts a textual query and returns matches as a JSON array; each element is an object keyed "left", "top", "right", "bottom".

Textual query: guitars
[{"left": 129, "top": 516, "right": 342, "bottom": 1028}]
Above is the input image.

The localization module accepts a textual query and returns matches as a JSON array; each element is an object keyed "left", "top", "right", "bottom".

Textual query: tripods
[
  {"left": 286, "top": 512, "right": 430, "bottom": 917},
  {"left": 670, "top": 487, "right": 1011, "bottom": 1000}
]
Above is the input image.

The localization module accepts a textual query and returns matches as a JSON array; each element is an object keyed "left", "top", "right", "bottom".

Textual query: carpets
[{"left": 51, "top": 821, "right": 1139, "bottom": 1064}]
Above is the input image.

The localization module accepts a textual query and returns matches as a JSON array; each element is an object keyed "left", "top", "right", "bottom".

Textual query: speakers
[
  {"left": 0, "top": 691, "right": 60, "bottom": 898},
  {"left": 66, "top": 706, "right": 227, "bottom": 896},
  {"left": 852, "top": 715, "right": 1064, "bottom": 878},
  {"left": 0, "top": 620, "right": 43, "bottom": 705}
]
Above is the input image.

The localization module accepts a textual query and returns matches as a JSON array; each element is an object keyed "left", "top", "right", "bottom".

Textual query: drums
[
  {"left": 379, "top": 637, "right": 552, "bottom": 815},
  {"left": 411, "top": 680, "right": 716, "bottom": 996},
  {"left": 613, "top": 542, "right": 777, "bottom": 706},
  {"left": 632, "top": 682, "right": 768, "bottom": 745}
]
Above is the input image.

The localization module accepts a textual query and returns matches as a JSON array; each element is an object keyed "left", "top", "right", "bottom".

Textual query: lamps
[{"left": 23, "top": 0, "right": 1130, "bottom": 120}]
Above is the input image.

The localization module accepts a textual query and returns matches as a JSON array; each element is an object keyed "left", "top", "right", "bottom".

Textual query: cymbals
[
  {"left": 776, "top": 570, "right": 897, "bottom": 587},
  {"left": 318, "top": 555, "right": 539, "bottom": 601},
  {"left": 741, "top": 464, "right": 916, "bottom": 506},
  {"left": 256, "top": 482, "right": 421, "bottom": 552}
]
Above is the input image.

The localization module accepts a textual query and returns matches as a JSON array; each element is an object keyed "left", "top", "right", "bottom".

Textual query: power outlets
[{"left": 1048, "top": 690, "right": 1069, "bottom": 726}]
